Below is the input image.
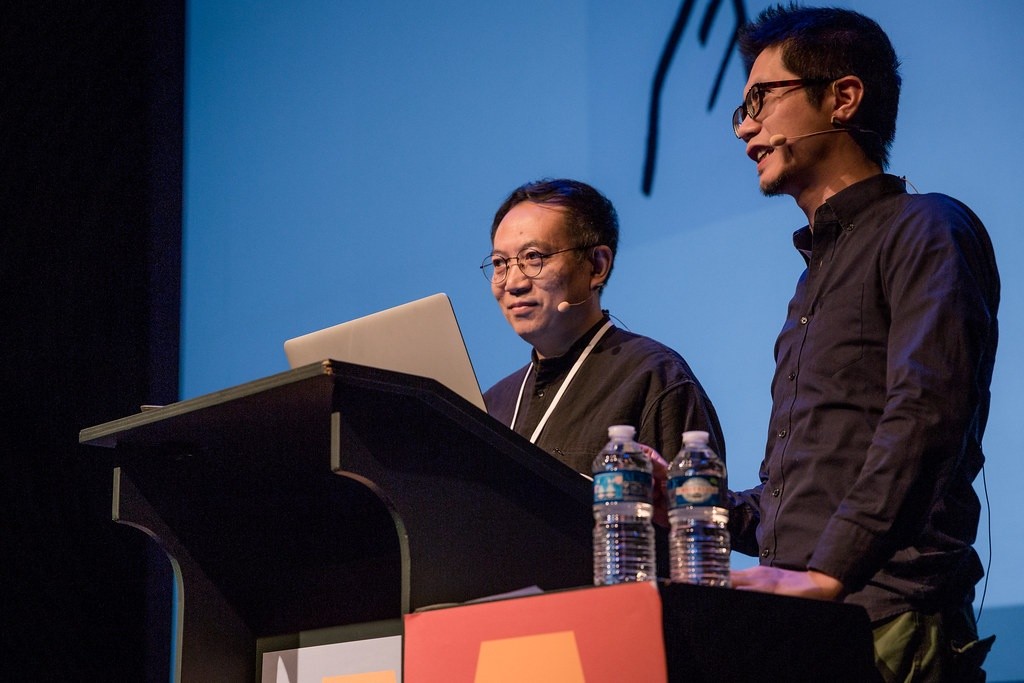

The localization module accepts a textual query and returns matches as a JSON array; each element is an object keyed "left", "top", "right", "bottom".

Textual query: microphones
[
  {"left": 769, "top": 129, "right": 845, "bottom": 147},
  {"left": 558, "top": 287, "right": 601, "bottom": 313}
]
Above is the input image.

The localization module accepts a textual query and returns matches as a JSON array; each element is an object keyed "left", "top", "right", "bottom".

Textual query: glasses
[
  {"left": 732, "top": 78, "right": 833, "bottom": 139},
  {"left": 480, "top": 246, "right": 587, "bottom": 284}
]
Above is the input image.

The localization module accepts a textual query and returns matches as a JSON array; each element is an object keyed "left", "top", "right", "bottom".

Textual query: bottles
[
  {"left": 592, "top": 425, "right": 656, "bottom": 587},
  {"left": 665, "top": 431, "right": 731, "bottom": 587}
]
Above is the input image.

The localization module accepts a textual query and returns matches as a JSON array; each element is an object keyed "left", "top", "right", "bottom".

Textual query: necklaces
[{"left": 507, "top": 319, "right": 613, "bottom": 444}]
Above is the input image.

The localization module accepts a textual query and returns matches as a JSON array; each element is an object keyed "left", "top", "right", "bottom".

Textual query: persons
[
  {"left": 480, "top": 178, "right": 727, "bottom": 538},
  {"left": 632, "top": 0, "right": 1001, "bottom": 683}
]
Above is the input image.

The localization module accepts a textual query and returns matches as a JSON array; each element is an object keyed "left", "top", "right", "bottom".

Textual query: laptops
[{"left": 284, "top": 291, "right": 488, "bottom": 414}]
